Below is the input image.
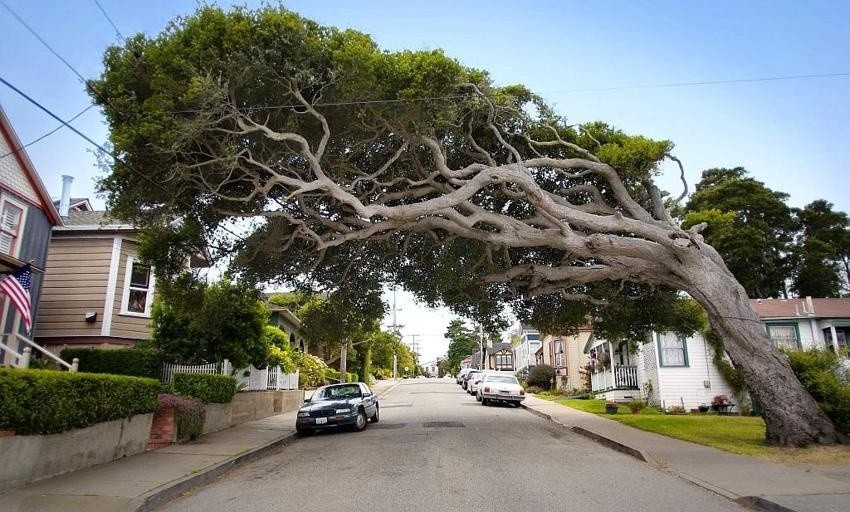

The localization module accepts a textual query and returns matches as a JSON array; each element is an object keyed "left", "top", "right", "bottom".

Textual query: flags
[{"left": 0, "top": 264, "right": 33, "bottom": 335}]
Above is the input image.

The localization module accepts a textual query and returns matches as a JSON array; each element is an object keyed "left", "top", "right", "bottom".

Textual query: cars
[
  {"left": 293, "top": 379, "right": 380, "bottom": 435},
  {"left": 415, "top": 371, "right": 455, "bottom": 379},
  {"left": 476, "top": 366, "right": 525, "bottom": 406},
  {"left": 456, "top": 368, "right": 483, "bottom": 396}
]
